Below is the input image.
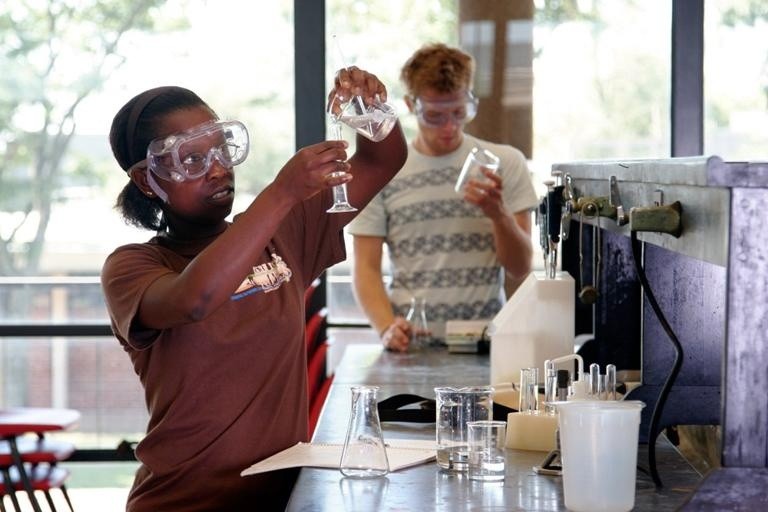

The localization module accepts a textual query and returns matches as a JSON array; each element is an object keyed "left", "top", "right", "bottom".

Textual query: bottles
[
  {"left": 400, "top": 297, "right": 431, "bottom": 357},
  {"left": 326, "top": 124, "right": 359, "bottom": 213},
  {"left": 340, "top": 386, "right": 390, "bottom": 478},
  {"left": 516, "top": 360, "right": 616, "bottom": 416}
]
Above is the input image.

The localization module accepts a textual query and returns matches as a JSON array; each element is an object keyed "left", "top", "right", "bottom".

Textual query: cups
[
  {"left": 465, "top": 417, "right": 507, "bottom": 478},
  {"left": 454, "top": 147, "right": 498, "bottom": 200},
  {"left": 540, "top": 398, "right": 646, "bottom": 511},
  {"left": 327, "top": 83, "right": 398, "bottom": 144},
  {"left": 432, "top": 382, "right": 495, "bottom": 473}
]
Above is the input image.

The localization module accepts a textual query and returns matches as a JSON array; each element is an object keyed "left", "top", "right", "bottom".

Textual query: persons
[
  {"left": 101, "top": 65, "right": 408, "bottom": 511},
  {"left": 347, "top": 42, "right": 539, "bottom": 353}
]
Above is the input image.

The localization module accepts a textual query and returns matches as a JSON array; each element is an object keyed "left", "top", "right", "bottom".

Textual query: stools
[{"left": 0, "top": 403, "right": 85, "bottom": 512}]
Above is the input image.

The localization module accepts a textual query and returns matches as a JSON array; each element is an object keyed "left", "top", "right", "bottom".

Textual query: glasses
[
  {"left": 405, "top": 91, "right": 480, "bottom": 129},
  {"left": 146, "top": 116, "right": 252, "bottom": 185}
]
